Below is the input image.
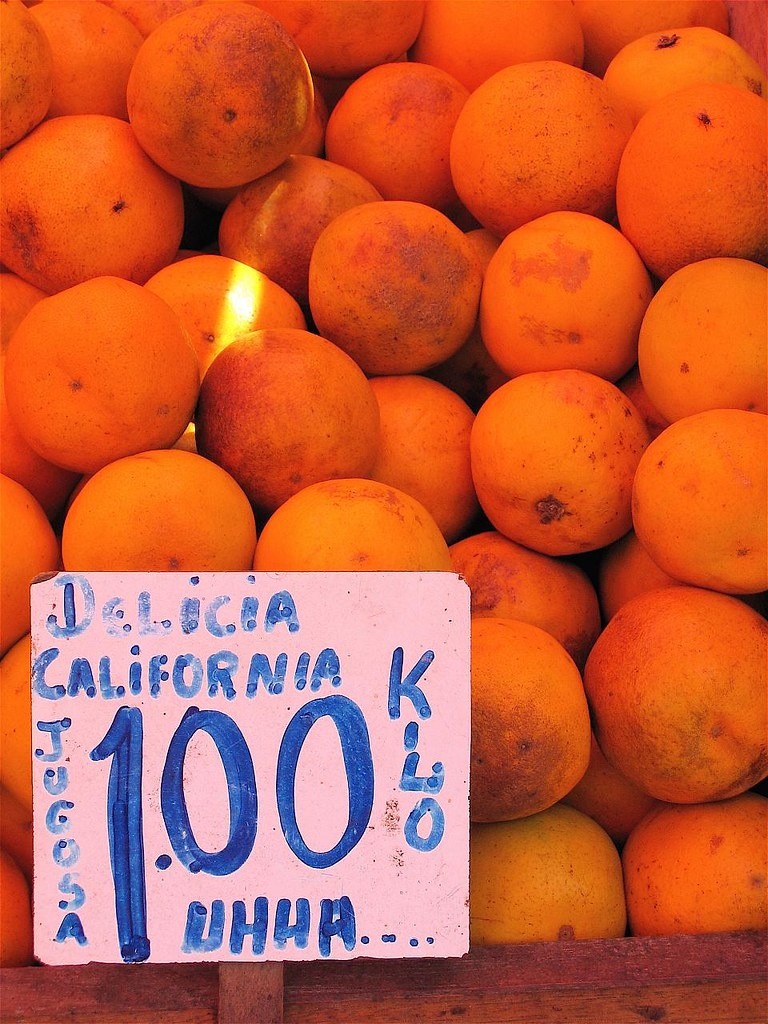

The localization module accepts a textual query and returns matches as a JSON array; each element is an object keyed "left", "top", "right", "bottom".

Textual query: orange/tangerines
[{"left": 0, "top": 0, "right": 768, "bottom": 968}]
